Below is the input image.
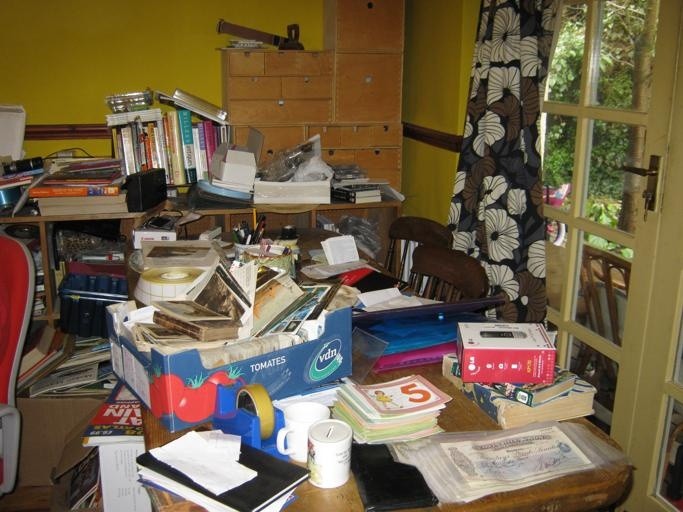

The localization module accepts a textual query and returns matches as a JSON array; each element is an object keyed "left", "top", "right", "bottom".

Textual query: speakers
[{"left": 127, "top": 168, "right": 167, "bottom": 212}]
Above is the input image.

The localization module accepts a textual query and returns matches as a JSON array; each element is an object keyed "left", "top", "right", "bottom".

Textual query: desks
[{"left": 113, "top": 280, "right": 635, "bottom": 510}]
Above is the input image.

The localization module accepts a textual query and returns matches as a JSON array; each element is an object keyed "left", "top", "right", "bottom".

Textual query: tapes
[{"left": 235, "top": 383, "right": 275, "bottom": 440}]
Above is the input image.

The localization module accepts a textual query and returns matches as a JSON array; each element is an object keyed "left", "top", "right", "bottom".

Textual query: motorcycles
[{"left": 543, "top": 177, "right": 569, "bottom": 251}]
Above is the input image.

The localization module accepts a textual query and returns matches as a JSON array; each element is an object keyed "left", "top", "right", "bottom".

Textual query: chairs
[
  {"left": 568, "top": 243, "right": 681, "bottom": 492},
  {"left": 0, "top": 232, "right": 36, "bottom": 499},
  {"left": 376, "top": 214, "right": 452, "bottom": 299},
  {"left": 405, "top": 246, "right": 487, "bottom": 318}
]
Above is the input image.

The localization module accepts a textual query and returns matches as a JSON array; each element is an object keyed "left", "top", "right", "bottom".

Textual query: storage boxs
[
  {"left": 454, "top": 318, "right": 558, "bottom": 388},
  {"left": 251, "top": 180, "right": 334, "bottom": 205},
  {"left": 215, "top": 121, "right": 265, "bottom": 187},
  {"left": 102, "top": 298, "right": 354, "bottom": 432},
  {"left": 51, "top": 252, "right": 131, "bottom": 339}
]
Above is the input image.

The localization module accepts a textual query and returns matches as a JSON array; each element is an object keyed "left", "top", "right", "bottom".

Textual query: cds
[{"left": 5, "top": 224, "right": 41, "bottom": 239}]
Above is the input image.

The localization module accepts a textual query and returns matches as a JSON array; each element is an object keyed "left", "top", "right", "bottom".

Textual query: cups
[{"left": 277, "top": 402, "right": 329, "bottom": 464}]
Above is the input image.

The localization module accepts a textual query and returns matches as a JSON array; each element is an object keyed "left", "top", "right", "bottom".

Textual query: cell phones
[{"left": 480, "top": 331, "right": 527, "bottom": 338}]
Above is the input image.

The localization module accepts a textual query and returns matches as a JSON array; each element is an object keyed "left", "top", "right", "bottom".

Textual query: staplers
[{"left": 199, "top": 227, "right": 223, "bottom": 242}]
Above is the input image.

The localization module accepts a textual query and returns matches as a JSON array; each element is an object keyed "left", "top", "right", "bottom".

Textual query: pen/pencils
[{"left": 231, "top": 208, "right": 266, "bottom": 245}]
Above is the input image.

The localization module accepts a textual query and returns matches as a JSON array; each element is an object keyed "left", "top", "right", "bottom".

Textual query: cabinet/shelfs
[
  {"left": 217, "top": 0, "right": 405, "bottom": 223},
  {"left": 2, "top": 187, "right": 401, "bottom": 324}
]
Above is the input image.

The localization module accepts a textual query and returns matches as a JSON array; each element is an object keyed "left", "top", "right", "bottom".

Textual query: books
[{"left": 0, "top": 87, "right": 631, "bottom": 511}]
